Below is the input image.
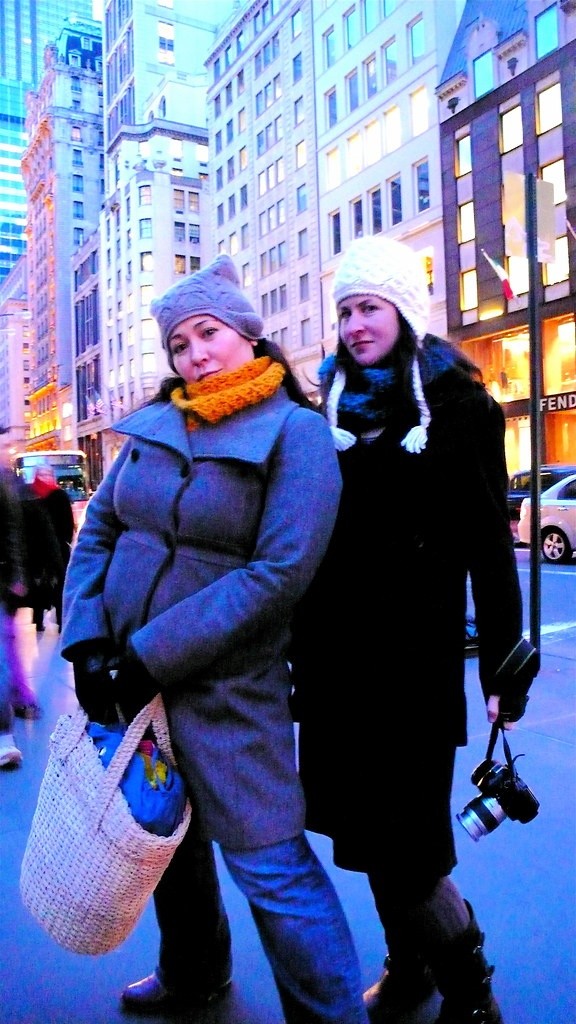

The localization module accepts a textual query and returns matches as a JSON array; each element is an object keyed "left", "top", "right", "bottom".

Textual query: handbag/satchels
[
  {"left": 76, "top": 723, "right": 186, "bottom": 835},
  {"left": 18, "top": 675, "right": 193, "bottom": 956}
]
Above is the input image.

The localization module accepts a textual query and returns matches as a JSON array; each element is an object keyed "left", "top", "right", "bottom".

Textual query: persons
[
  {"left": 0, "top": 255, "right": 368, "bottom": 1024},
  {"left": 288, "top": 235, "right": 541, "bottom": 1024}
]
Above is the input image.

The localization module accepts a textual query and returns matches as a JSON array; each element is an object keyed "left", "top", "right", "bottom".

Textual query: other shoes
[
  {"left": 120, "top": 962, "right": 233, "bottom": 1009},
  {"left": 19, "top": 701, "right": 42, "bottom": 719},
  {"left": 38, "top": 625, "right": 46, "bottom": 632},
  {"left": 0, "top": 735, "right": 22, "bottom": 767}
]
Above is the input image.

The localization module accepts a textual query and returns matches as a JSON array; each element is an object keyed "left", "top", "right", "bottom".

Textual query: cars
[
  {"left": 508, "top": 463, "right": 576, "bottom": 521},
  {"left": 517, "top": 474, "right": 576, "bottom": 565}
]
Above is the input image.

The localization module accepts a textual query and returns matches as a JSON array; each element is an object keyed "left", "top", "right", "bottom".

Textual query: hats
[
  {"left": 327, "top": 236, "right": 431, "bottom": 453},
  {"left": 150, "top": 254, "right": 265, "bottom": 373},
  {"left": 34, "top": 464, "right": 53, "bottom": 474}
]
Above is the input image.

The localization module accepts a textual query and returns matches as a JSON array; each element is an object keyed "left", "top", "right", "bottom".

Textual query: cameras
[{"left": 456, "top": 757, "right": 540, "bottom": 843}]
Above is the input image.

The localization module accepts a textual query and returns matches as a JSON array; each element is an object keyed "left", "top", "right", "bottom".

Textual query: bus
[{"left": 11, "top": 449, "right": 98, "bottom": 526}]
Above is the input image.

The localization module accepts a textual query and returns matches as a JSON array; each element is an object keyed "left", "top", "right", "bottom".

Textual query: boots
[
  {"left": 367, "top": 925, "right": 438, "bottom": 1024},
  {"left": 435, "top": 900, "right": 502, "bottom": 1024}
]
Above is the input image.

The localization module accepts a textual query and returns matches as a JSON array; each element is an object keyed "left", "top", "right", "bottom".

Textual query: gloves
[
  {"left": 75, "top": 645, "right": 119, "bottom": 724},
  {"left": 113, "top": 652, "right": 161, "bottom": 736}
]
